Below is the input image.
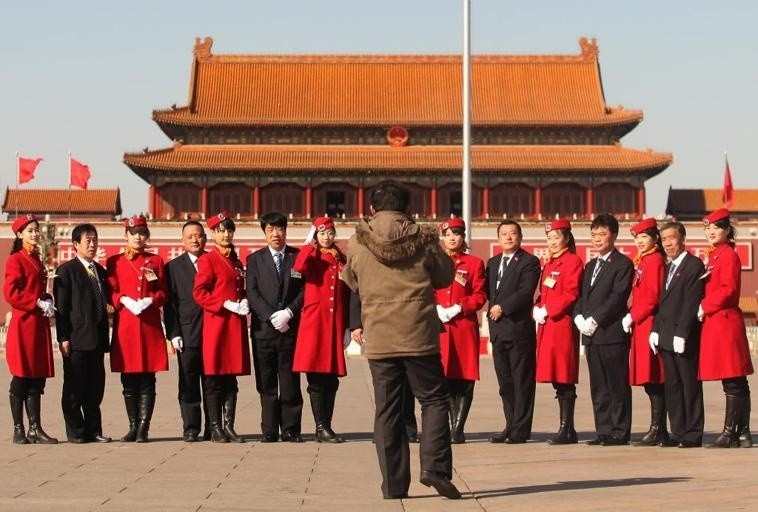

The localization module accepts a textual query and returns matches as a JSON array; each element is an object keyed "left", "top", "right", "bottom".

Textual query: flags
[
  {"left": 721, "top": 161, "right": 734, "bottom": 206},
  {"left": 70, "top": 158, "right": 91, "bottom": 189},
  {"left": 18, "top": 156, "right": 43, "bottom": 186}
]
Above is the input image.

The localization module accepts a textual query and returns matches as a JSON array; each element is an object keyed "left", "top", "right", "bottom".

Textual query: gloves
[
  {"left": 574, "top": 313, "right": 593, "bottom": 337},
  {"left": 673, "top": 336, "right": 685, "bottom": 354},
  {"left": 270, "top": 307, "right": 294, "bottom": 330},
  {"left": 121, "top": 296, "right": 141, "bottom": 316},
  {"left": 621, "top": 313, "right": 634, "bottom": 333},
  {"left": 171, "top": 336, "right": 184, "bottom": 353},
  {"left": 437, "top": 304, "right": 450, "bottom": 323},
  {"left": 36, "top": 298, "right": 58, "bottom": 317},
  {"left": 580, "top": 315, "right": 599, "bottom": 334},
  {"left": 697, "top": 304, "right": 704, "bottom": 323},
  {"left": 238, "top": 298, "right": 248, "bottom": 315},
  {"left": 278, "top": 323, "right": 291, "bottom": 334},
  {"left": 532, "top": 305, "right": 546, "bottom": 324},
  {"left": 223, "top": 298, "right": 250, "bottom": 316},
  {"left": 649, "top": 331, "right": 660, "bottom": 355},
  {"left": 533, "top": 306, "right": 548, "bottom": 323},
  {"left": 137, "top": 296, "right": 153, "bottom": 312},
  {"left": 445, "top": 304, "right": 462, "bottom": 320}
]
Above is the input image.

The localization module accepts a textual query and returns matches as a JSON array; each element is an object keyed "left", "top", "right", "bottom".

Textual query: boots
[
  {"left": 547, "top": 398, "right": 579, "bottom": 445},
  {"left": 325, "top": 391, "right": 345, "bottom": 442},
  {"left": 451, "top": 395, "right": 474, "bottom": 444},
  {"left": 703, "top": 394, "right": 743, "bottom": 448},
  {"left": 205, "top": 391, "right": 229, "bottom": 443},
  {"left": 25, "top": 393, "right": 59, "bottom": 444},
  {"left": 9, "top": 393, "right": 27, "bottom": 444},
  {"left": 120, "top": 393, "right": 137, "bottom": 442},
  {"left": 309, "top": 390, "right": 339, "bottom": 444},
  {"left": 736, "top": 389, "right": 752, "bottom": 448},
  {"left": 630, "top": 393, "right": 670, "bottom": 446},
  {"left": 222, "top": 391, "right": 244, "bottom": 443},
  {"left": 449, "top": 394, "right": 458, "bottom": 429},
  {"left": 135, "top": 392, "right": 156, "bottom": 443}
]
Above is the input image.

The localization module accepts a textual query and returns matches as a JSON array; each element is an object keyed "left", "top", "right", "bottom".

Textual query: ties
[
  {"left": 274, "top": 252, "right": 283, "bottom": 273},
  {"left": 495, "top": 256, "right": 510, "bottom": 291},
  {"left": 669, "top": 263, "right": 676, "bottom": 277},
  {"left": 89, "top": 264, "right": 104, "bottom": 323},
  {"left": 590, "top": 258, "right": 604, "bottom": 286}
]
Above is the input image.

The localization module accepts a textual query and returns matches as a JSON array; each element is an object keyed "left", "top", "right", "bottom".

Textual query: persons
[
  {"left": 696, "top": 207, "right": 754, "bottom": 449},
  {"left": 531, "top": 219, "right": 584, "bottom": 445},
  {"left": 3, "top": 213, "right": 59, "bottom": 445},
  {"left": 293, "top": 216, "right": 352, "bottom": 444},
  {"left": 52, "top": 223, "right": 113, "bottom": 443},
  {"left": 340, "top": 178, "right": 463, "bottom": 501},
  {"left": 485, "top": 219, "right": 542, "bottom": 444},
  {"left": 432, "top": 217, "right": 487, "bottom": 444},
  {"left": 192, "top": 211, "right": 251, "bottom": 444},
  {"left": 622, "top": 217, "right": 669, "bottom": 447},
  {"left": 647, "top": 222, "right": 705, "bottom": 449},
  {"left": 105, "top": 215, "right": 169, "bottom": 443},
  {"left": 571, "top": 214, "right": 635, "bottom": 446},
  {"left": 161, "top": 220, "right": 211, "bottom": 443},
  {"left": 245, "top": 211, "right": 305, "bottom": 445},
  {"left": 349, "top": 289, "right": 421, "bottom": 445}
]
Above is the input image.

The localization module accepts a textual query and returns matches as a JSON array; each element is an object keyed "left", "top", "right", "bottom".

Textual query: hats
[
  {"left": 440, "top": 217, "right": 466, "bottom": 230},
  {"left": 313, "top": 216, "right": 334, "bottom": 231},
  {"left": 124, "top": 216, "right": 147, "bottom": 227},
  {"left": 206, "top": 210, "right": 229, "bottom": 230},
  {"left": 630, "top": 217, "right": 657, "bottom": 237},
  {"left": 702, "top": 208, "right": 729, "bottom": 226},
  {"left": 11, "top": 211, "right": 36, "bottom": 233},
  {"left": 544, "top": 219, "right": 571, "bottom": 233}
]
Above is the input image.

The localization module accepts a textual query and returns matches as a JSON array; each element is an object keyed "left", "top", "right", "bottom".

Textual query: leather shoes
[
  {"left": 183, "top": 430, "right": 198, "bottom": 441},
  {"left": 600, "top": 436, "right": 628, "bottom": 446},
  {"left": 281, "top": 431, "right": 303, "bottom": 442},
  {"left": 407, "top": 434, "right": 421, "bottom": 443},
  {"left": 659, "top": 437, "right": 680, "bottom": 447},
  {"left": 504, "top": 436, "right": 527, "bottom": 444},
  {"left": 420, "top": 469, "right": 462, "bottom": 499},
  {"left": 94, "top": 434, "right": 112, "bottom": 443},
  {"left": 678, "top": 441, "right": 702, "bottom": 448},
  {"left": 489, "top": 434, "right": 505, "bottom": 443},
  {"left": 586, "top": 436, "right": 613, "bottom": 445},
  {"left": 67, "top": 436, "right": 86, "bottom": 443},
  {"left": 260, "top": 434, "right": 279, "bottom": 442}
]
